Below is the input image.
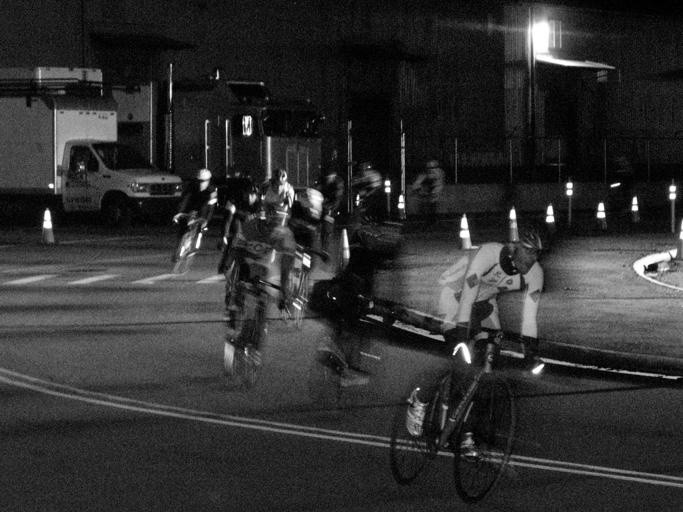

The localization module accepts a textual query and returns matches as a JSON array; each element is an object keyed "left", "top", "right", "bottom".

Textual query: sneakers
[
  {"left": 405, "top": 386, "right": 429, "bottom": 437},
  {"left": 460, "top": 432, "right": 479, "bottom": 457}
]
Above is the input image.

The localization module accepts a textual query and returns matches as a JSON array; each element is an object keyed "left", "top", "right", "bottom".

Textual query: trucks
[
  {"left": 113, "top": 60, "right": 325, "bottom": 206},
  {"left": 0, "top": 66, "right": 180, "bottom": 228}
]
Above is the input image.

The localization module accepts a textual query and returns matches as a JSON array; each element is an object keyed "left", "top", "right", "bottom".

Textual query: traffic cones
[
  {"left": 627, "top": 196, "right": 643, "bottom": 225},
  {"left": 590, "top": 201, "right": 609, "bottom": 233},
  {"left": 36, "top": 207, "right": 56, "bottom": 246},
  {"left": 544, "top": 204, "right": 558, "bottom": 236}
]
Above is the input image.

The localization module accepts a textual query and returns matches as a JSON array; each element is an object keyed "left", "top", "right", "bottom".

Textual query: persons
[
  {"left": 405, "top": 227, "right": 545, "bottom": 466},
  {"left": 314, "top": 194, "right": 404, "bottom": 390},
  {"left": 167, "top": 159, "right": 446, "bottom": 262},
  {"left": 218, "top": 202, "right": 296, "bottom": 381}
]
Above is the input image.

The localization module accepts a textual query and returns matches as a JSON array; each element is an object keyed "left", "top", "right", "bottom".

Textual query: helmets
[
  {"left": 196, "top": 169, "right": 212, "bottom": 181},
  {"left": 517, "top": 227, "right": 544, "bottom": 250}
]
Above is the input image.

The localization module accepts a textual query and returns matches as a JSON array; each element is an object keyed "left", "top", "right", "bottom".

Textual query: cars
[{"left": 186, "top": 172, "right": 259, "bottom": 217}]
